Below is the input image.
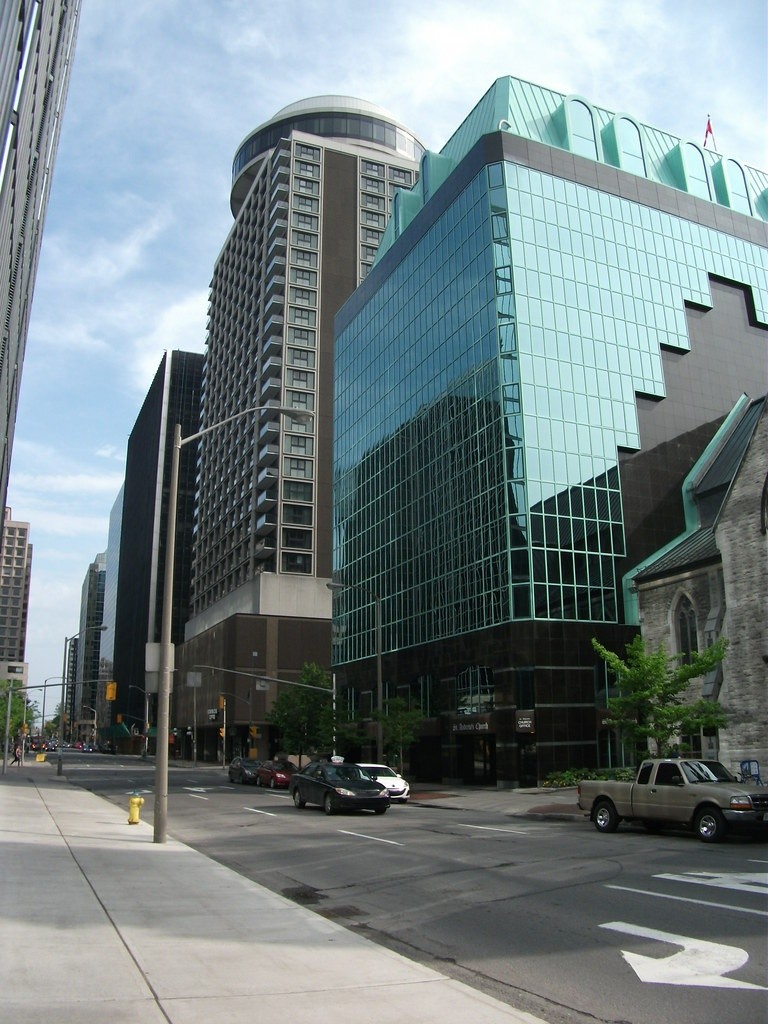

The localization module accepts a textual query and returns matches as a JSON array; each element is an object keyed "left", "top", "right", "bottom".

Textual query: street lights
[
  {"left": 325, "top": 582, "right": 384, "bottom": 765},
  {"left": 127, "top": 684, "right": 150, "bottom": 756},
  {"left": 22, "top": 689, "right": 43, "bottom": 762},
  {"left": 41, "top": 676, "right": 68, "bottom": 739},
  {"left": 150, "top": 407, "right": 318, "bottom": 843},
  {"left": 57, "top": 624, "right": 109, "bottom": 776},
  {"left": 82, "top": 705, "right": 97, "bottom": 745}
]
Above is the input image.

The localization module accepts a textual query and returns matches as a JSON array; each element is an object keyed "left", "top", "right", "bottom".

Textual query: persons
[
  {"left": 10, "top": 745, "right": 21, "bottom": 767},
  {"left": 349, "top": 771, "right": 362, "bottom": 780}
]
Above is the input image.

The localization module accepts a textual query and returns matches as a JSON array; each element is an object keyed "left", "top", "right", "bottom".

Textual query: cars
[
  {"left": 352, "top": 763, "right": 410, "bottom": 803},
  {"left": 227, "top": 757, "right": 265, "bottom": 784},
  {"left": 255, "top": 760, "right": 302, "bottom": 789},
  {"left": 288, "top": 761, "right": 391, "bottom": 815},
  {"left": 31, "top": 738, "right": 111, "bottom": 754}
]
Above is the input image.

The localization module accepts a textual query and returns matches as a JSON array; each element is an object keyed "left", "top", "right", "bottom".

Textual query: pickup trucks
[{"left": 575, "top": 758, "right": 768, "bottom": 843}]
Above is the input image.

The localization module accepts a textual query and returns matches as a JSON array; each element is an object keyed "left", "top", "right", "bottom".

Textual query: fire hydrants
[{"left": 128, "top": 794, "right": 144, "bottom": 823}]
